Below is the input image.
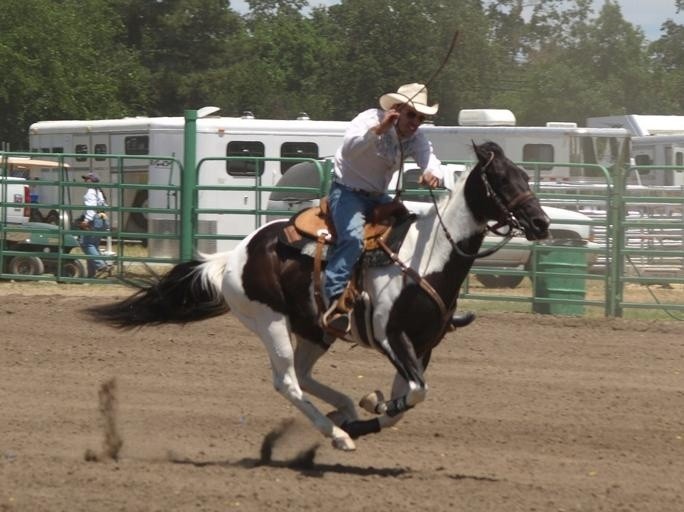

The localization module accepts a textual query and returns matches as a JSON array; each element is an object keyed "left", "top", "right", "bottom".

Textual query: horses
[{"left": 74, "top": 138, "right": 553, "bottom": 452}]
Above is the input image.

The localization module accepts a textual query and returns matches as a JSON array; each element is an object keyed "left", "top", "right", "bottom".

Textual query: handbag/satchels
[{"left": 93, "top": 213, "right": 110, "bottom": 230}]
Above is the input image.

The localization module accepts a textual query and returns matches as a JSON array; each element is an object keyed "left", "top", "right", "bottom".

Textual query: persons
[
  {"left": 73, "top": 174, "right": 114, "bottom": 281},
  {"left": 317, "top": 83, "right": 475, "bottom": 345}
]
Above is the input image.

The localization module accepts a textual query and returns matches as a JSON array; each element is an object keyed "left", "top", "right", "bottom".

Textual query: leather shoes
[
  {"left": 450, "top": 311, "right": 476, "bottom": 327},
  {"left": 322, "top": 314, "right": 348, "bottom": 345}
]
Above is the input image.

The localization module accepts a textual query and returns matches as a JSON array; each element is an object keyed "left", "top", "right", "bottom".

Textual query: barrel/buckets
[{"left": 532, "top": 245, "right": 589, "bottom": 316}]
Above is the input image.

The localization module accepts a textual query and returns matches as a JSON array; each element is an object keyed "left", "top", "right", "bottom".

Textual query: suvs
[{"left": 262, "top": 158, "right": 601, "bottom": 291}]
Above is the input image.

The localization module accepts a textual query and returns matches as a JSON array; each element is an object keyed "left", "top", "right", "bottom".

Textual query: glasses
[{"left": 406, "top": 111, "right": 427, "bottom": 121}]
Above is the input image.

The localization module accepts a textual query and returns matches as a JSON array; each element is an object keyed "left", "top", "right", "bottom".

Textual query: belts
[{"left": 352, "top": 188, "right": 384, "bottom": 197}]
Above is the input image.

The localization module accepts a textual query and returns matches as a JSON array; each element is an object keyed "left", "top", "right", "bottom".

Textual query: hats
[
  {"left": 81, "top": 174, "right": 100, "bottom": 182},
  {"left": 379, "top": 83, "right": 440, "bottom": 115}
]
